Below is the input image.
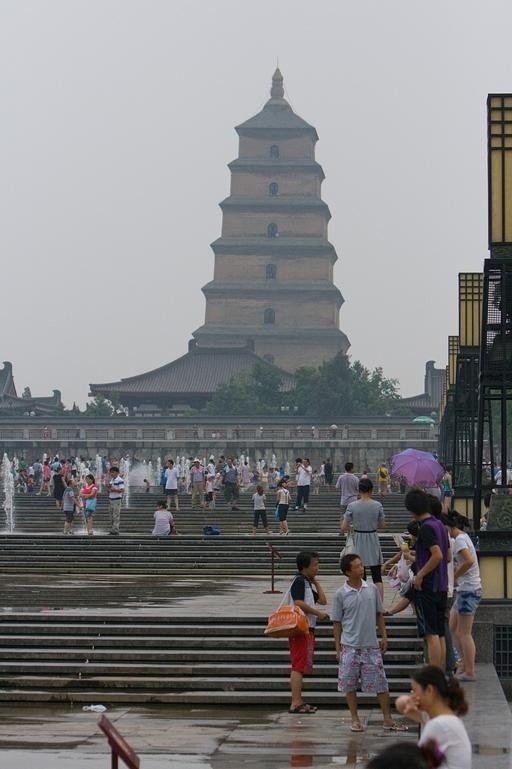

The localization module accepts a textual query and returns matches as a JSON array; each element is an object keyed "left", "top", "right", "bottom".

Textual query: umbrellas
[{"left": 411, "top": 415, "right": 436, "bottom": 439}]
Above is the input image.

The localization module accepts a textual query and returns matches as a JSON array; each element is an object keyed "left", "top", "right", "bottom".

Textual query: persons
[
  {"left": 274, "top": 549, "right": 333, "bottom": 715},
  {"left": 14, "top": 421, "right": 512, "bottom": 540},
  {"left": 339, "top": 477, "right": 387, "bottom": 608},
  {"left": 393, "top": 663, "right": 475, "bottom": 768},
  {"left": 363, "top": 737, "right": 448, "bottom": 769},
  {"left": 438, "top": 508, "right": 489, "bottom": 684},
  {"left": 328, "top": 552, "right": 411, "bottom": 733},
  {"left": 405, "top": 487, "right": 453, "bottom": 671},
  {"left": 382, "top": 539, "right": 420, "bottom": 616}
]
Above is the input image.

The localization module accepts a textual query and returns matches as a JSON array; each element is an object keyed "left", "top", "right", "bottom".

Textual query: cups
[{"left": 400, "top": 543, "right": 409, "bottom": 559}]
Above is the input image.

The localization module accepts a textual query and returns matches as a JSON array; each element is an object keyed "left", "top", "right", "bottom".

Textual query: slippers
[
  {"left": 382, "top": 722, "right": 409, "bottom": 731},
  {"left": 289, "top": 702, "right": 317, "bottom": 714},
  {"left": 349, "top": 724, "right": 363, "bottom": 731}
]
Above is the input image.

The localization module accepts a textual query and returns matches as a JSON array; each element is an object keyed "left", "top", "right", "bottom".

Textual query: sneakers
[
  {"left": 454, "top": 670, "right": 476, "bottom": 680},
  {"left": 295, "top": 505, "right": 308, "bottom": 510},
  {"left": 88, "top": 529, "right": 93, "bottom": 535},
  {"left": 107, "top": 532, "right": 119, "bottom": 535},
  {"left": 63, "top": 529, "right": 75, "bottom": 534}
]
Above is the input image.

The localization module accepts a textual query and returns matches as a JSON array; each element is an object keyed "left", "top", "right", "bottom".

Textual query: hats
[
  {"left": 193, "top": 458, "right": 200, "bottom": 461},
  {"left": 207, "top": 474, "right": 213, "bottom": 478}
]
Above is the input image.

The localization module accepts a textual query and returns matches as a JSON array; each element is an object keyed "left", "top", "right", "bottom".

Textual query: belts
[
  {"left": 108, "top": 498, "right": 121, "bottom": 500},
  {"left": 353, "top": 529, "right": 376, "bottom": 533}
]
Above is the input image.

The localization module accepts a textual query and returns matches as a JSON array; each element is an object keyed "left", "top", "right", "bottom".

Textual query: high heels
[
  {"left": 278, "top": 530, "right": 290, "bottom": 535},
  {"left": 35, "top": 491, "right": 41, "bottom": 496},
  {"left": 46, "top": 492, "right": 51, "bottom": 496}
]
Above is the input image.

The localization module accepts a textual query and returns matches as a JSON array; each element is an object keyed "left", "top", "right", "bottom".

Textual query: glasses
[
  {"left": 409, "top": 689, "right": 430, "bottom": 695},
  {"left": 193, "top": 462, "right": 200, "bottom": 463}
]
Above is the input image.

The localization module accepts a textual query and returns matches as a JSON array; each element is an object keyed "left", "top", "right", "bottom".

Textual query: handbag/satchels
[
  {"left": 380, "top": 471, "right": 386, "bottom": 479},
  {"left": 42, "top": 476, "right": 50, "bottom": 482},
  {"left": 265, "top": 604, "right": 310, "bottom": 638},
  {"left": 86, "top": 498, "right": 97, "bottom": 512}
]
